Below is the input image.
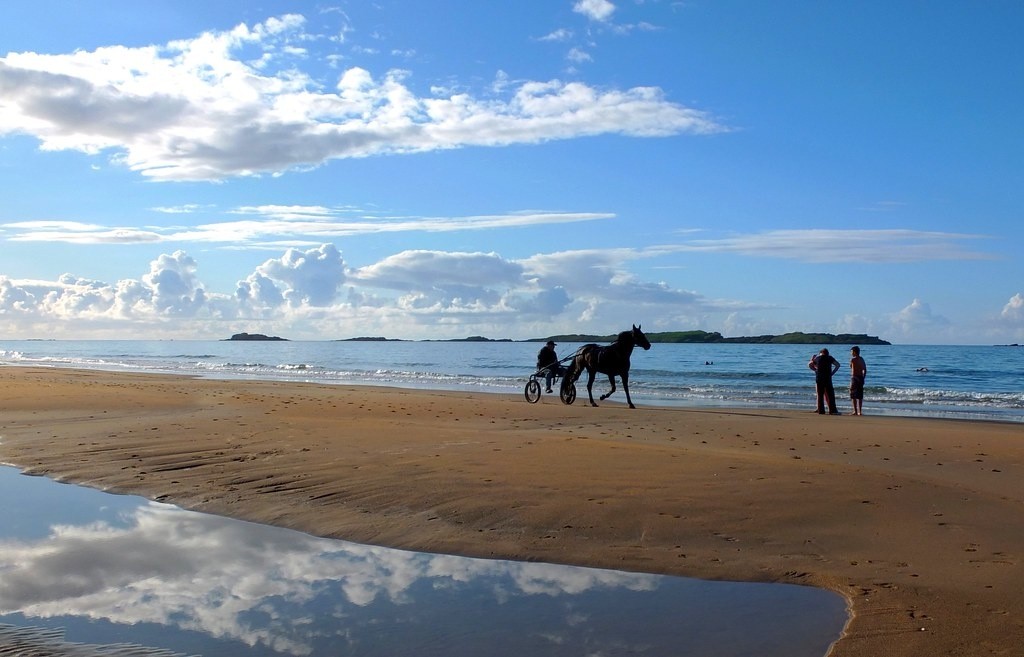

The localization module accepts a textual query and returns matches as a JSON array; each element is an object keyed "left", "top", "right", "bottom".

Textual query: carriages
[{"left": 525, "top": 324, "right": 651, "bottom": 409}]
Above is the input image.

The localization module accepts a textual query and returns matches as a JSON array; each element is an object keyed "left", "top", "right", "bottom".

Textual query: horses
[{"left": 564, "top": 323, "right": 652, "bottom": 410}]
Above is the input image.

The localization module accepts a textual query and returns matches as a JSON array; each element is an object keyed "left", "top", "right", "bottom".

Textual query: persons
[
  {"left": 849, "top": 346, "right": 867, "bottom": 416},
  {"left": 809, "top": 348, "right": 842, "bottom": 415},
  {"left": 537, "top": 341, "right": 570, "bottom": 395}
]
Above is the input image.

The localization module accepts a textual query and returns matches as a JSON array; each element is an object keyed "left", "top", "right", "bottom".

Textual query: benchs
[{"left": 536, "top": 363, "right": 570, "bottom": 377}]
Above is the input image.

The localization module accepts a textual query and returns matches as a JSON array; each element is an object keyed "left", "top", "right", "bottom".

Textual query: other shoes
[
  {"left": 851, "top": 412, "right": 861, "bottom": 416},
  {"left": 546, "top": 390, "right": 552, "bottom": 393},
  {"left": 814, "top": 409, "right": 819, "bottom": 413},
  {"left": 830, "top": 412, "right": 841, "bottom": 415}
]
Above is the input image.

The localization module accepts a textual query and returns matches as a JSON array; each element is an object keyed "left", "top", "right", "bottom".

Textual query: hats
[{"left": 546, "top": 340, "right": 557, "bottom": 345}]
